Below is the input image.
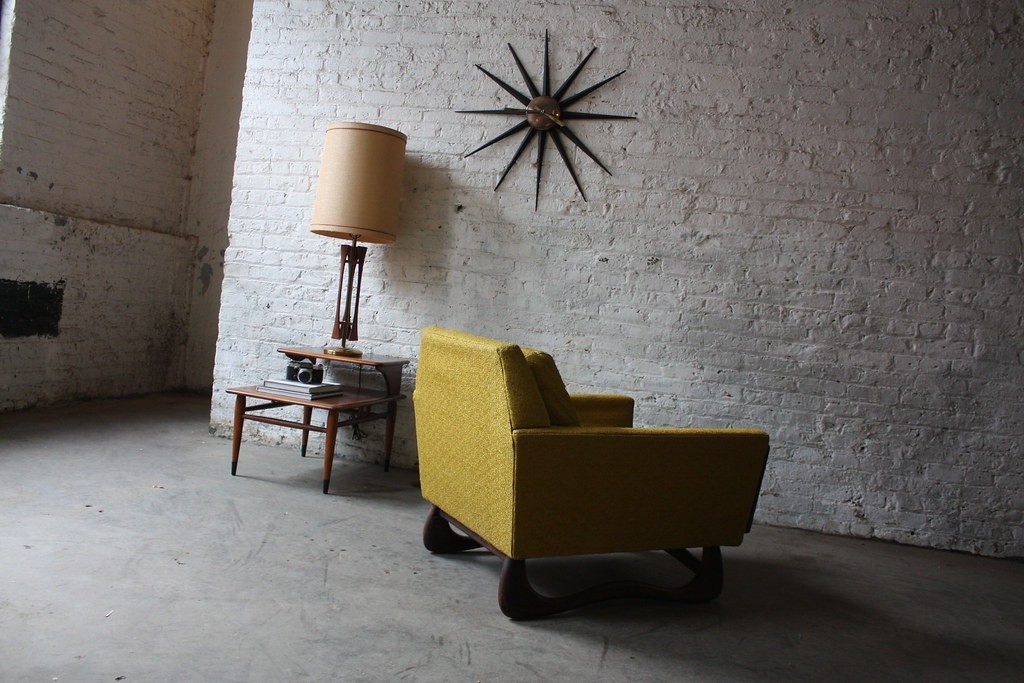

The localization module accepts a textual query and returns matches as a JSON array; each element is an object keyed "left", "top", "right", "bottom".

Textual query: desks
[{"left": 226, "top": 347, "right": 410, "bottom": 494}]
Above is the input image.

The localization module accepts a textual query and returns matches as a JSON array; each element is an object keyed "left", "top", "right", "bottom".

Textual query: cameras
[{"left": 286, "top": 361, "right": 324, "bottom": 385}]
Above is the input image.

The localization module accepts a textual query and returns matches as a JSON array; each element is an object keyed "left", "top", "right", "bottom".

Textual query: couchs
[{"left": 412, "top": 324, "right": 770, "bottom": 621}]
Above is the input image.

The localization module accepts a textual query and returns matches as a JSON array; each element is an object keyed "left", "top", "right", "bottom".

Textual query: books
[{"left": 256, "top": 380, "right": 344, "bottom": 400}]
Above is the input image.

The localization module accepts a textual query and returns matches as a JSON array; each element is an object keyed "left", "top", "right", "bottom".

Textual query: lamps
[{"left": 309, "top": 121, "right": 408, "bottom": 357}]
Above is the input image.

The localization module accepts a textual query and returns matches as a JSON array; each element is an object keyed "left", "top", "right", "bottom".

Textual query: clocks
[{"left": 455, "top": 28, "right": 638, "bottom": 212}]
